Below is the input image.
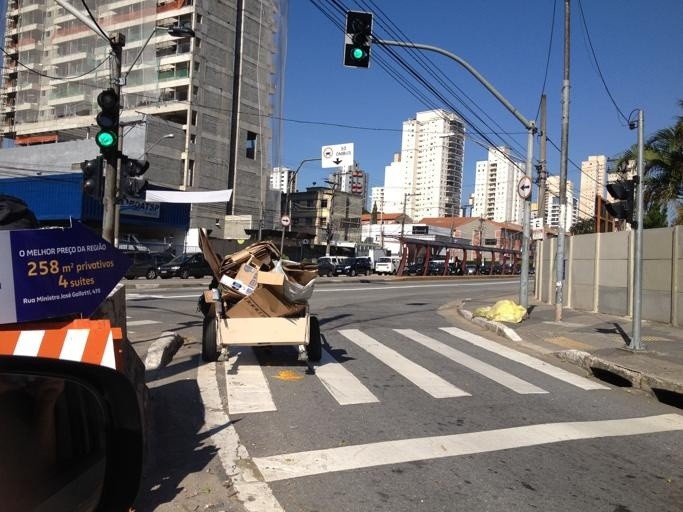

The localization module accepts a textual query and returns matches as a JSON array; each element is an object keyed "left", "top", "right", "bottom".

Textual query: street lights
[
  {"left": 114, "top": 124, "right": 174, "bottom": 252},
  {"left": 101, "top": 24, "right": 195, "bottom": 252}
]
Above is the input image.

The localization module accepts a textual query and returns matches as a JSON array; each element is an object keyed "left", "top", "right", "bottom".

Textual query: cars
[
  {"left": 118, "top": 233, "right": 223, "bottom": 280},
  {"left": 305, "top": 255, "right": 535, "bottom": 278}
]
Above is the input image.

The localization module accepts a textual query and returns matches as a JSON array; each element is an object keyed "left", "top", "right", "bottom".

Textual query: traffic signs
[{"left": 322, "top": 143, "right": 355, "bottom": 169}]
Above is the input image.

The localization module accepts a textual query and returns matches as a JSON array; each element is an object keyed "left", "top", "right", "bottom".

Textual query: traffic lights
[
  {"left": 119, "top": 154, "right": 151, "bottom": 203},
  {"left": 350, "top": 170, "right": 365, "bottom": 195},
  {"left": 96, "top": 87, "right": 119, "bottom": 153},
  {"left": 340, "top": 9, "right": 374, "bottom": 69}
]
